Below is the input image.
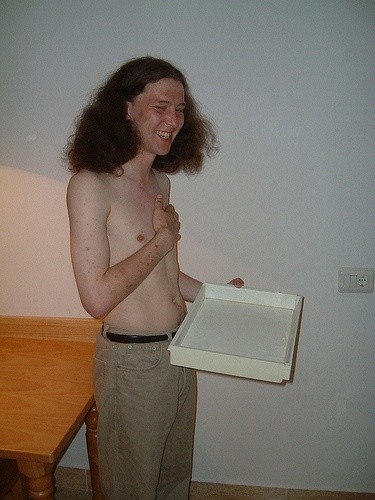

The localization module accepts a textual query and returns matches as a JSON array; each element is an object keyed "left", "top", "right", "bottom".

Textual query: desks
[{"left": 0, "top": 313, "right": 115, "bottom": 500}]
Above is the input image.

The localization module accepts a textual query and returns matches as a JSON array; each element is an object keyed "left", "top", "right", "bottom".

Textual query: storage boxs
[{"left": 165, "top": 281, "right": 304, "bottom": 386}]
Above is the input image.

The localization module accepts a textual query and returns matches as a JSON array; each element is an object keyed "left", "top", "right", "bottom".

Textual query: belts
[{"left": 100, "top": 330, "right": 177, "bottom": 343}]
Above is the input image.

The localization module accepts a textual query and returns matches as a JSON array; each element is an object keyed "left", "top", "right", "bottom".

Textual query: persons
[{"left": 64, "top": 52, "right": 246, "bottom": 499}]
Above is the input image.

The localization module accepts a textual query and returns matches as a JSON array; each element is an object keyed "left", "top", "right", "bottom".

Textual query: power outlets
[{"left": 337, "top": 267, "right": 374, "bottom": 293}]
